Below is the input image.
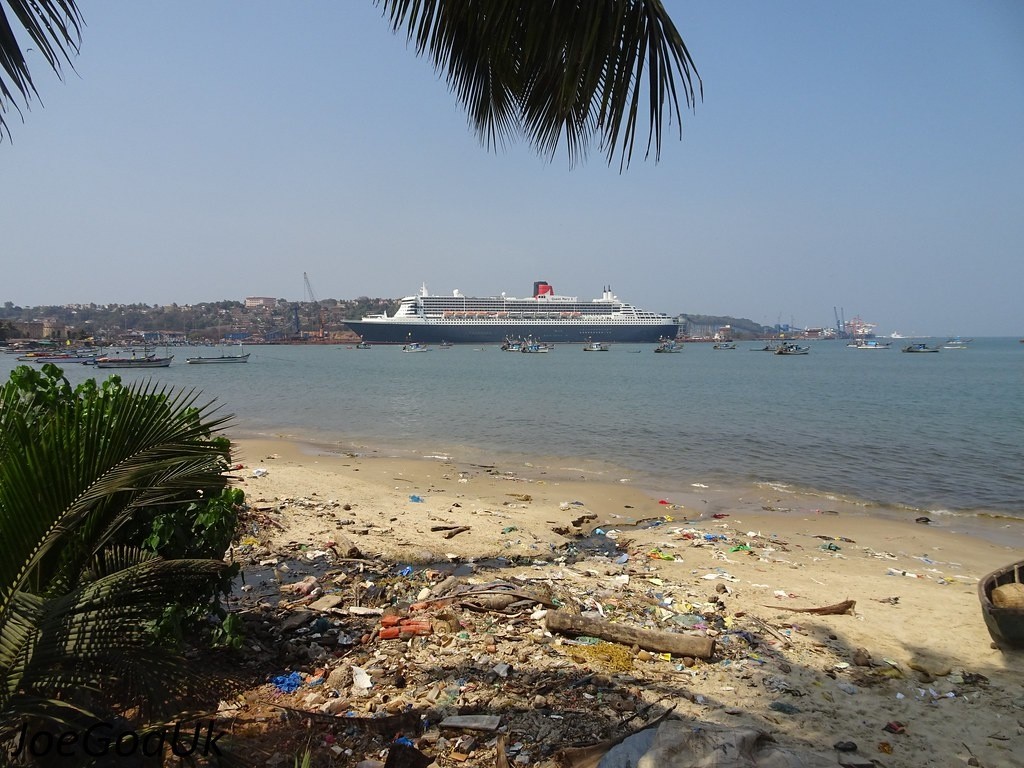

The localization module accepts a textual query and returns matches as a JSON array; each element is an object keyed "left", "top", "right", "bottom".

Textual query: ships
[{"left": 340, "top": 279, "right": 681, "bottom": 343}]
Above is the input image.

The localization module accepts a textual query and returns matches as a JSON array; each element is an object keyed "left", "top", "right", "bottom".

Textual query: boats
[
  {"left": 823, "top": 306, "right": 907, "bottom": 349},
  {"left": 356, "top": 340, "right": 372, "bottom": 349},
  {"left": 774, "top": 340, "right": 812, "bottom": 355},
  {"left": 583, "top": 337, "right": 609, "bottom": 351},
  {"left": 978, "top": 558, "right": 1024, "bottom": 651},
  {"left": 186, "top": 342, "right": 252, "bottom": 364},
  {"left": 712, "top": 340, "right": 736, "bottom": 350},
  {"left": 902, "top": 341, "right": 939, "bottom": 353},
  {"left": 402, "top": 333, "right": 428, "bottom": 353},
  {"left": 0, "top": 336, "right": 176, "bottom": 369},
  {"left": 500, "top": 332, "right": 557, "bottom": 353},
  {"left": 748, "top": 344, "right": 776, "bottom": 352},
  {"left": 654, "top": 335, "right": 684, "bottom": 353},
  {"left": 440, "top": 340, "right": 453, "bottom": 347},
  {"left": 943, "top": 335, "right": 974, "bottom": 350}
]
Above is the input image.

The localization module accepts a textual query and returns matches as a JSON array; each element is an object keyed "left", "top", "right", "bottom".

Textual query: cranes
[{"left": 303, "top": 271, "right": 326, "bottom": 330}]
